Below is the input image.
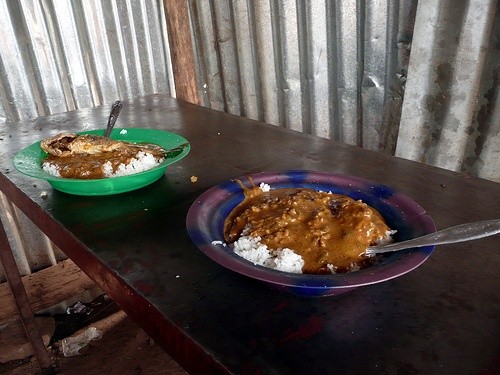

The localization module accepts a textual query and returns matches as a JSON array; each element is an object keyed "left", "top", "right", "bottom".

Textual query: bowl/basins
[
  {"left": 12, "top": 128, "right": 192, "bottom": 196},
  {"left": 185, "top": 169, "right": 438, "bottom": 297}
]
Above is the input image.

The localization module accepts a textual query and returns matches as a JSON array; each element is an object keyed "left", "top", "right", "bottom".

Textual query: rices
[
  {"left": 211, "top": 181, "right": 397, "bottom": 275},
  {"left": 41, "top": 128, "right": 166, "bottom": 179}
]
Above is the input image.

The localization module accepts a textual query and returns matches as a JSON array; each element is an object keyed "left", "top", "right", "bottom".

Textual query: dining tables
[{"left": 0, "top": 94, "right": 500, "bottom": 375}]
